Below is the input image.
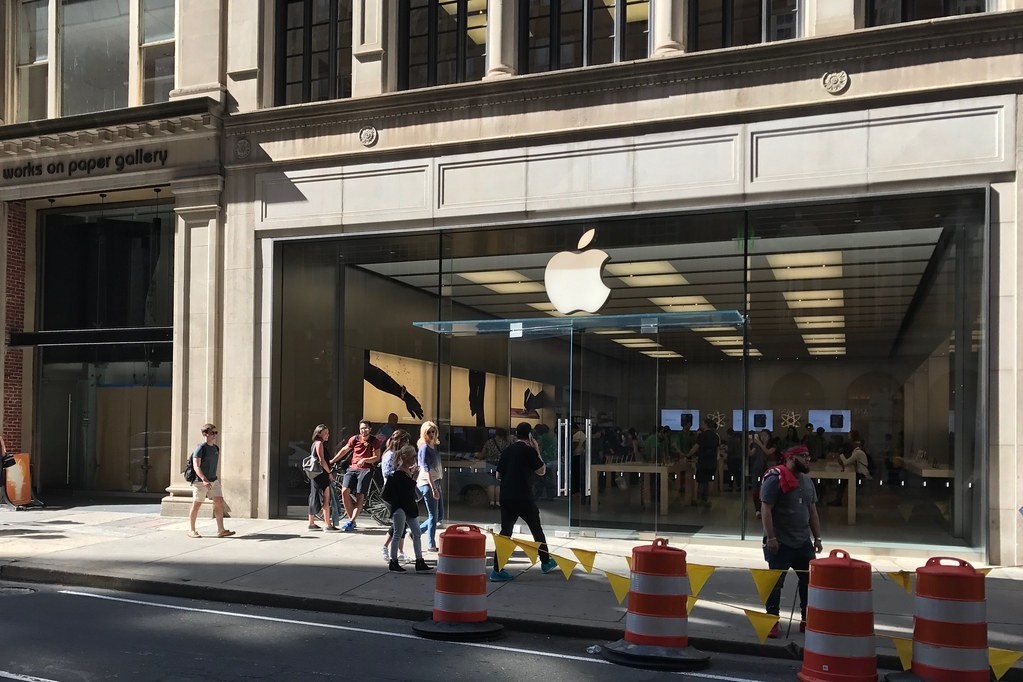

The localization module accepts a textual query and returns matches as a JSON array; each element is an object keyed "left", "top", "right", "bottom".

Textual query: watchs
[{"left": 400, "top": 385, "right": 407, "bottom": 398}]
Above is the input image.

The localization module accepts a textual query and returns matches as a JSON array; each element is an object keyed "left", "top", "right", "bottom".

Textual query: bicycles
[{"left": 315, "top": 463, "right": 393, "bottom": 526}]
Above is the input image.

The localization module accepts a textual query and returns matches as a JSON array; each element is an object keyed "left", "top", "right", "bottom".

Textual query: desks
[
  {"left": 793, "top": 453, "right": 857, "bottom": 527},
  {"left": 590, "top": 453, "right": 726, "bottom": 514}
]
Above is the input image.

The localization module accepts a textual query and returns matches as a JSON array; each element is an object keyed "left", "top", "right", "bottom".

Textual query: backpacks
[
  {"left": 184, "top": 444, "right": 219, "bottom": 482},
  {"left": 856, "top": 449, "right": 877, "bottom": 476}
]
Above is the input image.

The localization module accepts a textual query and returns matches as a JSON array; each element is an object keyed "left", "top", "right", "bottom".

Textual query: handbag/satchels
[
  {"left": 2, "top": 453, "right": 16, "bottom": 468},
  {"left": 614, "top": 475, "right": 627, "bottom": 490},
  {"left": 302, "top": 444, "right": 323, "bottom": 479},
  {"left": 340, "top": 434, "right": 359, "bottom": 471},
  {"left": 380, "top": 474, "right": 399, "bottom": 509}
]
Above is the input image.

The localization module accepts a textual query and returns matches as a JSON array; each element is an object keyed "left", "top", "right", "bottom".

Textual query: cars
[
  {"left": 288, "top": 441, "right": 311, "bottom": 493},
  {"left": 439, "top": 452, "right": 496, "bottom": 508},
  {"left": 125, "top": 432, "right": 170, "bottom": 485}
]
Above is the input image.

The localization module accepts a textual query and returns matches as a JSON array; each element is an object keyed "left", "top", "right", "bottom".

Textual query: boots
[
  {"left": 388, "top": 559, "right": 407, "bottom": 572},
  {"left": 415, "top": 558, "right": 434, "bottom": 572}
]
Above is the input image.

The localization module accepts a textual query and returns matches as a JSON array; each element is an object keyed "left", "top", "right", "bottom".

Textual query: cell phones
[
  {"left": 754, "top": 434, "right": 758, "bottom": 440},
  {"left": 529, "top": 432, "right": 533, "bottom": 444},
  {"left": 749, "top": 435, "right": 752, "bottom": 442}
]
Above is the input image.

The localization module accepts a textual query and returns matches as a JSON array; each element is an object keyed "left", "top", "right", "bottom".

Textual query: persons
[
  {"left": 0, "top": 435, "right": 6, "bottom": 476},
  {"left": 489, "top": 422, "right": 557, "bottom": 581},
  {"left": 380, "top": 445, "right": 435, "bottom": 573},
  {"left": 379, "top": 413, "right": 399, "bottom": 454},
  {"left": 329, "top": 419, "right": 381, "bottom": 531},
  {"left": 589, "top": 418, "right": 875, "bottom": 518},
  {"left": 411, "top": 421, "right": 445, "bottom": 553},
  {"left": 364, "top": 350, "right": 424, "bottom": 420},
  {"left": 881, "top": 431, "right": 905, "bottom": 482},
  {"left": 381, "top": 430, "right": 414, "bottom": 561},
  {"left": 757, "top": 440, "right": 822, "bottom": 639},
  {"left": 307, "top": 424, "right": 341, "bottom": 533},
  {"left": 468, "top": 369, "right": 486, "bottom": 426},
  {"left": 188, "top": 424, "right": 236, "bottom": 539},
  {"left": 479, "top": 424, "right": 585, "bottom": 509}
]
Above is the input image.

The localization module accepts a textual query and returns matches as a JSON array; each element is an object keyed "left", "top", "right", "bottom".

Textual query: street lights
[{"left": 135, "top": 344, "right": 161, "bottom": 493}]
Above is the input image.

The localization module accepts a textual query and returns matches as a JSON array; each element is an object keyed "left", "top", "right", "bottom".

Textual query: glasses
[
  {"left": 210, "top": 431, "right": 217, "bottom": 435},
  {"left": 792, "top": 453, "right": 812, "bottom": 459},
  {"left": 427, "top": 431, "right": 434, "bottom": 434}
]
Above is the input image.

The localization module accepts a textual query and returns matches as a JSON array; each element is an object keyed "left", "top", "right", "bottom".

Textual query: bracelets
[
  {"left": 815, "top": 537, "right": 821, "bottom": 542},
  {"left": 767, "top": 537, "right": 776, "bottom": 542}
]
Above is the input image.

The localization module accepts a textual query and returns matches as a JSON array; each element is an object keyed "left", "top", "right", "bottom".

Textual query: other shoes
[
  {"left": 768, "top": 621, "right": 780, "bottom": 637},
  {"left": 428, "top": 546, "right": 439, "bottom": 552},
  {"left": 496, "top": 502, "right": 501, "bottom": 508},
  {"left": 188, "top": 531, "right": 202, "bottom": 538},
  {"left": 800, "top": 621, "right": 806, "bottom": 632},
  {"left": 398, "top": 553, "right": 410, "bottom": 561},
  {"left": 756, "top": 510, "right": 762, "bottom": 519},
  {"left": 409, "top": 531, "right": 413, "bottom": 539},
  {"left": 381, "top": 546, "right": 390, "bottom": 561},
  {"left": 826, "top": 499, "right": 842, "bottom": 506},
  {"left": 489, "top": 501, "right": 495, "bottom": 509},
  {"left": 217, "top": 530, "right": 235, "bottom": 538}
]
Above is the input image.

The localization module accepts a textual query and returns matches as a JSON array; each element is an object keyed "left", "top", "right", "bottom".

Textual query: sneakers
[
  {"left": 490, "top": 569, "right": 514, "bottom": 581},
  {"left": 308, "top": 525, "right": 322, "bottom": 530},
  {"left": 323, "top": 526, "right": 341, "bottom": 532},
  {"left": 541, "top": 557, "right": 557, "bottom": 573},
  {"left": 342, "top": 521, "right": 355, "bottom": 531}
]
